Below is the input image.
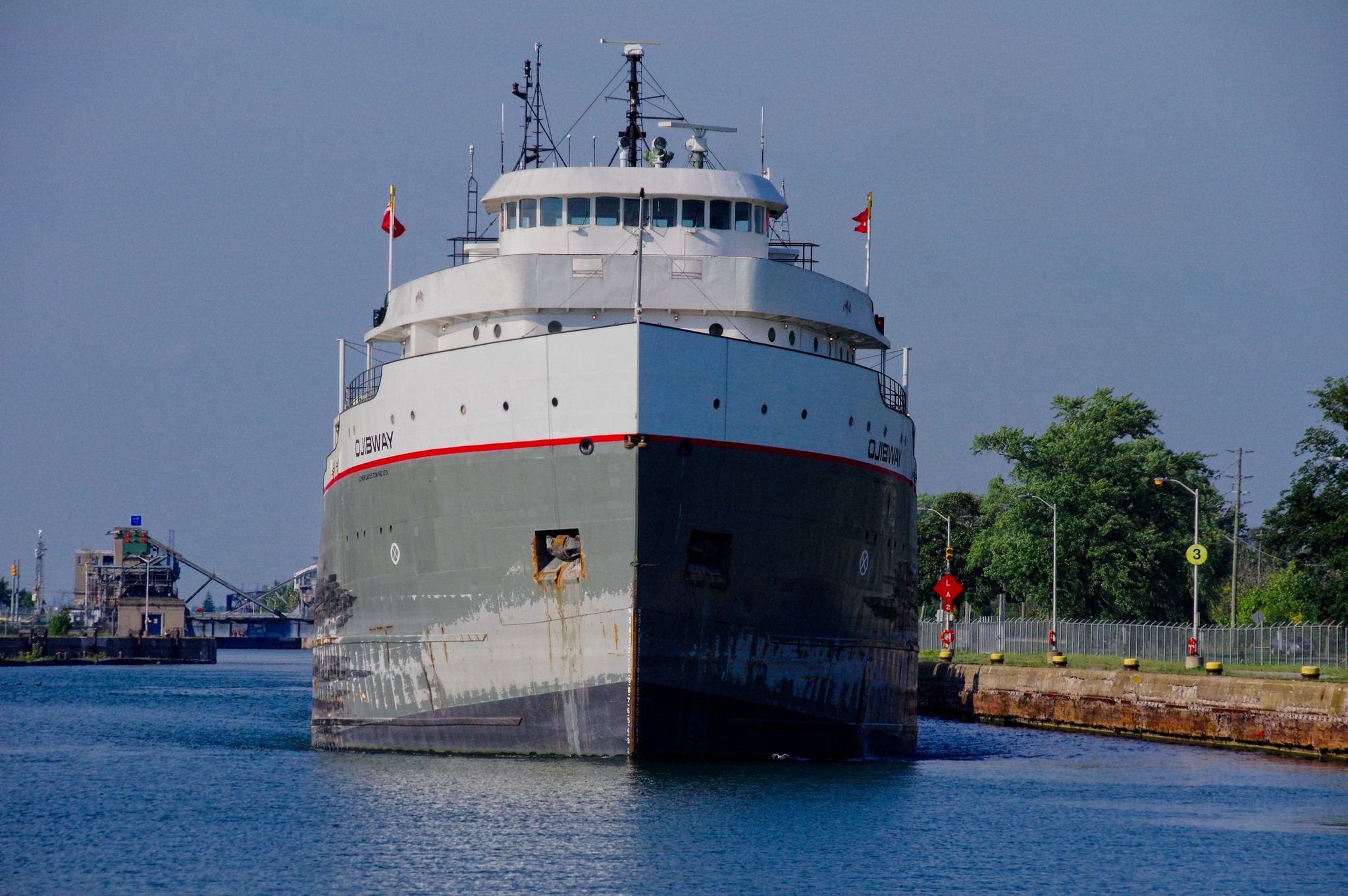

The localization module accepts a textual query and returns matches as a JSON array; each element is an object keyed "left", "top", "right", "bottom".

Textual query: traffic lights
[
  {"left": 11, "top": 565, "right": 17, "bottom": 576},
  {"left": 945, "top": 547, "right": 953, "bottom": 562}
]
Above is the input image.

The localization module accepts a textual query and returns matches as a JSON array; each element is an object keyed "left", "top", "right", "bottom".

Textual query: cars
[
  {"left": 1087, "top": 636, "right": 1108, "bottom": 648},
  {"left": 1269, "top": 635, "right": 1317, "bottom": 657},
  {"left": 957, "top": 630, "right": 978, "bottom": 644}
]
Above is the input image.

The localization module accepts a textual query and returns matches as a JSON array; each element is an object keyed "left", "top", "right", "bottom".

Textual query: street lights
[
  {"left": 918, "top": 507, "right": 954, "bottom": 650},
  {"left": 1017, "top": 494, "right": 1057, "bottom": 652},
  {"left": 129, "top": 554, "right": 167, "bottom": 630},
  {"left": 1153, "top": 476, "right": 1198, "bottom": 656}
]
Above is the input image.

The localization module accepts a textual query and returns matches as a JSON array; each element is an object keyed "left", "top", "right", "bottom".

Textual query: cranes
[{"left": 45, "top": 589, "right": 73, "bottom": 610}]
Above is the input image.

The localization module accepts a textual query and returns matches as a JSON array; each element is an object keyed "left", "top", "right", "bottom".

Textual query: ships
[{"left": 310, "top": 38, "right": 917, "bottom": 766}]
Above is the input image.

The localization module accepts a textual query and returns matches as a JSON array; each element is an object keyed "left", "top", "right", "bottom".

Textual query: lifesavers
[
  {"left": 1049, "top": 630, "right": 1054, "bottom": 645},
  {"left": 940, "top": 630, "right": 955, "bottom": 643},
  {"left": 1188, "top": 638, "right": 1195, "bottom": 655}
]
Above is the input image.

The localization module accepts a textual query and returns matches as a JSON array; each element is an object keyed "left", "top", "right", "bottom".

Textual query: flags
[
  {"left": 852, "top": 206, "right": 868, "bottom": 233},
  {"left": 381, "top": 203, "right": 405, "bottom": 239}
]
